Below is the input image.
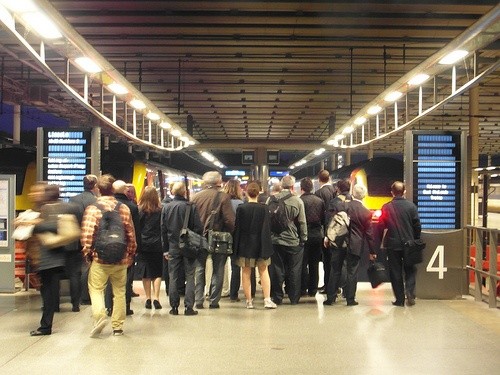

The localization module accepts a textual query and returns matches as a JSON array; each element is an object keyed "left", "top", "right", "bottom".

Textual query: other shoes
[
  {"left": 263, "top": 299, "right": 277, "bottom": 309},
  {"left": 131, "top": 292, "right": 139, "bottom": 297},
  {"left": 195, "top": 305, "right": 219, "bottom": 309},
  {"left": 229, "top": 287, "right": 327, "bottom": 302},
  {"left": 107, "top": 309, "right": 133, "bottom": 315},
  {"left": 72, "top": 307, "right": 80, "bottom": 312},
  {"left": 244, "top": 298, "right": 253, "bottom": 308}
]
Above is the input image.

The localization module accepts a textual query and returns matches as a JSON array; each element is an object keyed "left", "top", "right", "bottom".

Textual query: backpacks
[
  {"left": 328, "top": 212, "right": 351, "bottom": 249},
  {"left": 95, "top": 202, "right": 124, "bottom": 264},
  {"left": 268, "top": 194, "right": 295, "bottom": 234}
]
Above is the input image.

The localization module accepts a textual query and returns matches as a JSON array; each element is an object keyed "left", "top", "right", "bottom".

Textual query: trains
[
  {"left": 0, "top": 143, "right": 205, "bottom": 219},
  {"left": 293, "top": 154, "right": 406, "bottom": 225}
]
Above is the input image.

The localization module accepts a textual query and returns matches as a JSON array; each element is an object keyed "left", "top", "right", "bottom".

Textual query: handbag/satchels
[
  {"left": 403, "top": 240, "right": 425, "bottom": 267},
  {"left": 368, "top": 261, "right": 385, "bottom": 289},
  {"left": 12, "top": 211, "right": 39, "bottom": 240},
  {"left": 179, "top": 229, "right": 208, "bottom": 259},
  {"left": 208, "top": 231, "right": 233, "bottom": 257},
  {"left": 36, "top": 214, "right": 83, "bottom": 250}
]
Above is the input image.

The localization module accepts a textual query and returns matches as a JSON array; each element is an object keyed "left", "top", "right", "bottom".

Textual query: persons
[
  {"left": 370, "top": 181, "right": 421, "bottom": 306},
  {"left": 10, "top": 169, "right": 376, "bottom": 338}
]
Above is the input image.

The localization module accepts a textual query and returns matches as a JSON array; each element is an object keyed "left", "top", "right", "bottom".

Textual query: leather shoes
[
  {"left": 169, "top": 307, "right": 179, "bottom": 315},
  {"left": 184, "top": 308, "right": 198, "bottom": 316}
]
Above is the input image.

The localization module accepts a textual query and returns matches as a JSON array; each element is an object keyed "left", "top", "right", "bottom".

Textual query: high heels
[{"left": 145, "top": 300, "right": 162, "bottom": 309}]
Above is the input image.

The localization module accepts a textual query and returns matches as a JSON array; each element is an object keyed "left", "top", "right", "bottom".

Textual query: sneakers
[
  {"left": 113, "top": 330, "right": 123, "bottom": 336},
  {"left": 347, "top": 300, "right": 358, "bottom": 306},
  {"left": 90, "top": 317, "right": 109, "bottom": 336},
  {"left": 30, "top": 327, "right": 51, "bottom": 336},
  {"left": 392, "top": 299, "right": 415, "bottom": 307},
  {"left": 324, "top": 300, "right": 335, "bottom": 305}
]
